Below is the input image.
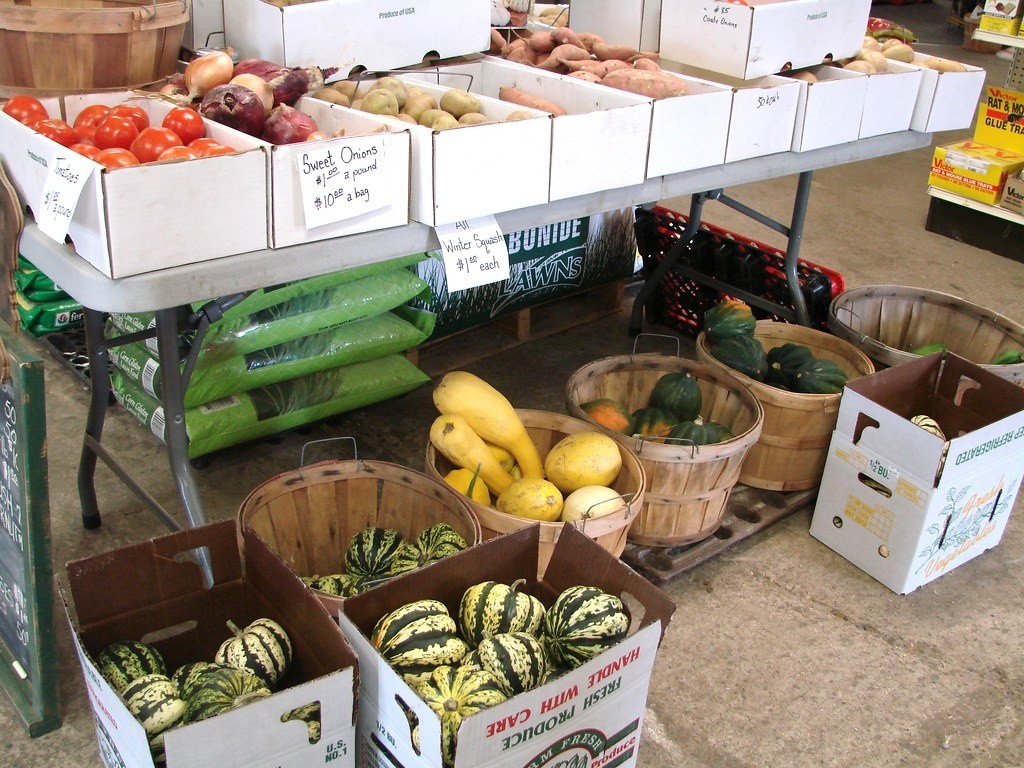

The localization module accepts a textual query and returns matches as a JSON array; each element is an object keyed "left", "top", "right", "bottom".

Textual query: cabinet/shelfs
[{"left": 924, "top": 29, "right": 1024, "bottom": 265}]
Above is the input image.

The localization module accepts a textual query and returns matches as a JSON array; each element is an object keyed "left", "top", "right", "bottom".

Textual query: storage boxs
[
  {"left": 56, "top": 519, "right": 360, "bottom": 768},
  {"left": 338, "top": 521, "right": 678, "bottom": 768},
  {"left": 979, "top": 0, "right": 1024, "bottom": 39},
  {"left": 808, "top": 351, "right": 1024, "bottom": 595},
  {"left": 0, "top": 0, "right": 987, "bottom": 282},
  {"left": 927, "top": 85, "right": 1024, "bottom": 215}
]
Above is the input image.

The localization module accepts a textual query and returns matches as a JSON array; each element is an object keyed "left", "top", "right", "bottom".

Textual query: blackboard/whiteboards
[{"left": 0, "top": 319, "right": 58, "bottom": 737}]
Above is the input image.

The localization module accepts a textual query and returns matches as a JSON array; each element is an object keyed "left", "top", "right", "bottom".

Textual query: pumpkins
[{"left": 92, "top": 294, "right": 943, "bottom": 768}]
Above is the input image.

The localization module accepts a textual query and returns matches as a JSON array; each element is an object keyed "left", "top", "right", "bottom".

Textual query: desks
[{"left": 12, "top": 133, "right": 934, "bottom": 586}]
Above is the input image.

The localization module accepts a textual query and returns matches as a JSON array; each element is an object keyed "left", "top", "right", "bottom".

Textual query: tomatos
[{"left": 3, "top": 96, "right": 238, "bottom": 170}]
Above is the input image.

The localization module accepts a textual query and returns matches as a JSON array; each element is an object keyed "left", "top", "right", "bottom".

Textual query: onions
[{"left": 160, "top": 52, "right": 334, "bottom": 142}]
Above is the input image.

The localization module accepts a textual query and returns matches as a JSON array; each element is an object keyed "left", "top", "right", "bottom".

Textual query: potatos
[
  {"left": 789, "top": 36, "right": 966, "bottom": 83},
  {"left": 491, "top": 8, "right": 688, "bottom": 116},
  {"left": 312, "top": 76, "right": 535, "bottom": 130}
]
